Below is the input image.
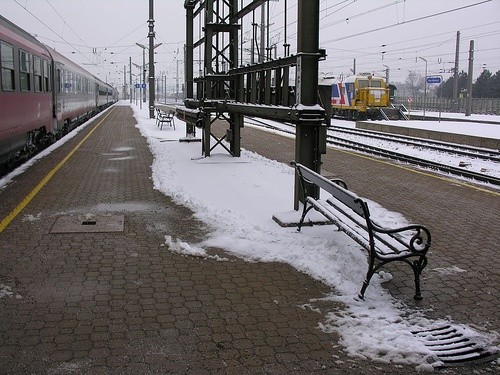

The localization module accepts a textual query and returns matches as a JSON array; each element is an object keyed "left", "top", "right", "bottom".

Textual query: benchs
[
  {"left": 289, "top": 160, "right": 430, "bottom": 302},
  {"left": 154, "top": 110, "right": 176, "bottom": 130}
]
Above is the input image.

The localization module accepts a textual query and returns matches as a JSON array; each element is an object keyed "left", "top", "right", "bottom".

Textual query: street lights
[
  {"left": 133, "top": 42, "right": 163, "bottom": 109},
  {"left": 418, "top": 56, "right": 427, "bottom": 120}
]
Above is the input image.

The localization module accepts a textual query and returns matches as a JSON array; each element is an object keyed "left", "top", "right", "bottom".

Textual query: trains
[
  {"left": 0, "top": 15, "right": 119, "bottom": 170},
  {"left": 289, "top": 72, "right": 410, "bottom": 121}
]
